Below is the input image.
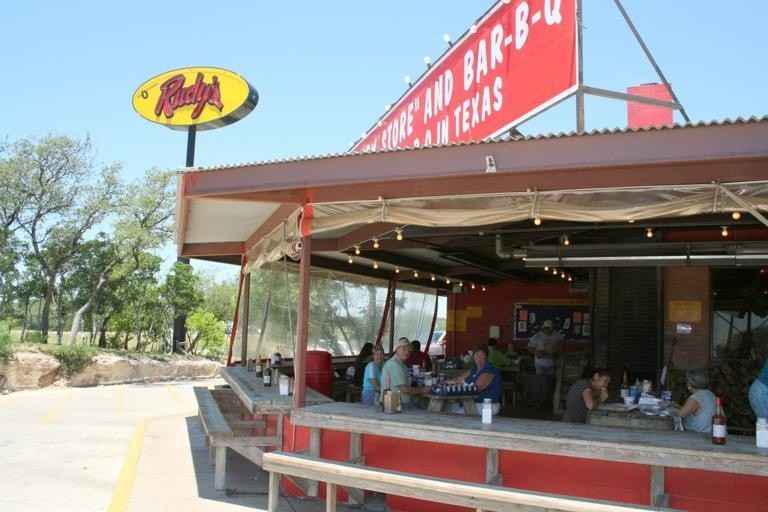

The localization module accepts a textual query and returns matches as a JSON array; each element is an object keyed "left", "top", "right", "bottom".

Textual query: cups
[
  {"left": 622, "top": 379, "right": 653, "bottom": 407},
  {"left": 422, "top": 371, "right": 478, "bottom": 393},
  {"left": 662, "top": 390, "right": 674, "bottom": 403}
]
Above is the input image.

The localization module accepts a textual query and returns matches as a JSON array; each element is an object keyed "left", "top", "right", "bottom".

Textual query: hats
[
  {"left": 395, "top": 339, "right": 413, "bottom": 351},
  {"left": 542, "top": 320, "right": 553, "bottom": 329}
]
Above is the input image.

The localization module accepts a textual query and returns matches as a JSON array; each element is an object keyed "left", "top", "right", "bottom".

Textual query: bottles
[
  {"left": 755, "top": 416, "right": 768, "bottom": 448},
  {"left": 262, "top": 359, "right": 272, "bottom": 387},
  {"left": 272, "top": 345, "right": 281, "bottom": 364},
  {"left": 620, "top": 366, "right": 629, "bottom": 403},
  {"left": 279, "top": 374, "right": 289, "bottom": 396},
  {"left": 256, "top": 354, "right": 263, "bottom": 377},
  {"left": 710, "top": 397, "right": 726, "bottom": 444},
  {"left": 273, "top": 368, "right": 280, "bottom": 385},
  {"left": 482, "top": 398, "right": 492, "bottom": 425}
]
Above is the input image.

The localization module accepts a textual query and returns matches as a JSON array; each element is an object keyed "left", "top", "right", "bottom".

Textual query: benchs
[
  {"left": 264, "top": 448, "right": 768, "bottom": 512},
  {"left": 193, "top": 386, "right": 277, "bottom": 490},
  {"left": 345, "top": 384, "right": 361, "bottom": 401}
]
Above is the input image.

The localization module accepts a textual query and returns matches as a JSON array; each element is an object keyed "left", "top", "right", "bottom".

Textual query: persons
[
  {"left": 747, "top": 361, "right": 768, "bottom": 422},
  {"left": 350, "top": 334, "right": 521, "bottom": 419},
  {"left": 559, "top": 364, "right": 612, "bottom": 426},
  {"left": 525, "top": 318, "right": 566, "bottom": 406},
  {"left": 656, "top": 366, "right": 730, "bottom": 435}
]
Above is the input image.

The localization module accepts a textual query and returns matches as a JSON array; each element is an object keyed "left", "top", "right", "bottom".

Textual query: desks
[{"left": 290, "top": 403, "right": 768, "bottom": 512}]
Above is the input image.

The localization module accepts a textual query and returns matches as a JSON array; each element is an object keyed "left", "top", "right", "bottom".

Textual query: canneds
[{"left": 432, "top": 384, "right": 478, "bottom": 393}]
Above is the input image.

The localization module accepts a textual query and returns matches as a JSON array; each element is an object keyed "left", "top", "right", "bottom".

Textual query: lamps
[
  {"left": 346, "top": 255, "right": 485, "bottom": 291},
  {"left": 354, "top": 229, "right": 403, "bottom": 254},
  {"left": 646, "top": 228, "right": 653, "bottom": 237},
  {"left": 721, "top": 226, "right": 728, "bottom": 236}
]
